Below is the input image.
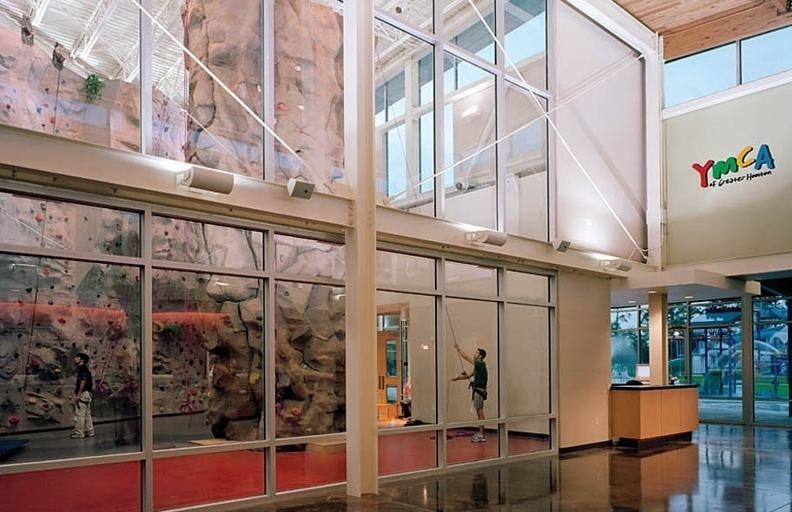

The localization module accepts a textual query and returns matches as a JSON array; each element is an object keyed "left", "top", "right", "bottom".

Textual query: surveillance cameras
[{"left": 455, "top": 172, "right": 470, "bottom": 194}]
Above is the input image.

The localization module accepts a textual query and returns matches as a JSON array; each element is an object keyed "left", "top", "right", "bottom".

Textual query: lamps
[
  {"left": 175, "top": 165, "right": 236, "bottom": 198},
  {"left": 462, "top": 228, "right": 507, "bottom": 248}
]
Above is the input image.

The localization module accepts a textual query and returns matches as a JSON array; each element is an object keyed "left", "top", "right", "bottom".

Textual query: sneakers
[
  {"left": 70, "top": 430, "right": 95, "bottom": 438},
  {"left": 471, "top": 432, "right": 487, "bottom": 442}
]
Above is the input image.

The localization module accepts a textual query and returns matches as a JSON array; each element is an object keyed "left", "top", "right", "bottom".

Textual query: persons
[
  {"left": 453, "top": 343, "right": 488, "bottom": 444},
  {"left": 454, "top": 472, "right": 490, "bottom": 512},
  {"left": 70, "top": 352, "right": 96, "bottom": 439},
  {"left": 110, "top": 397, "right": 140, "bottom": 446}
]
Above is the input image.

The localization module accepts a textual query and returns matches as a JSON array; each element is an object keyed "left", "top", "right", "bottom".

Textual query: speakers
[
  {"left": 551, "top": 239, "right": 571, "bottom": 252},
  {"left": 286, "top": 176, "right": 315, "bottom": 201}
]
